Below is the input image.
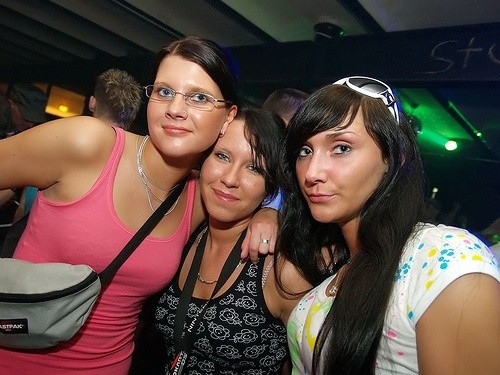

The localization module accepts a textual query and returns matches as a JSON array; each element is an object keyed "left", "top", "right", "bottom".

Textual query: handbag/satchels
[{"left": 0, "top": 177, "right": 190, "bottom": 349}]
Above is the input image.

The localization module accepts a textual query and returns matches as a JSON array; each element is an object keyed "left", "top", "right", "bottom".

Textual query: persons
[
  {"left": 0, "top": 37, "right": 283, "bottom": 375},
  {"left": 274, "top": 76, "right": 500, "bottom": 375},
  {"left": 0, "top": 81, "right": 48, "bottom": 203},
  {"left": 89, "top": 69, "right": 142, "bottom": 130},
  {"left": 150, "top": 101, "right": 317, "bottom": 375}
]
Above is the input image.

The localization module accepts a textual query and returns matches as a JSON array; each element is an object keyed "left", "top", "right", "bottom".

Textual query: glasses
[
  {"left": 333, "top": 76, "right": 399, "bottom": 125},
  {"left": 144, "top": 85, "right": 232, "bottom": 112}
]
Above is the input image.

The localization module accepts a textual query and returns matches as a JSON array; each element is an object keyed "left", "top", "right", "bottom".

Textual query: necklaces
[
  {"left": 329, "top": 257, "right": 352, "bottom": 294},
  {"left": 136, "top": 135, "right": 190, "bottom": 217},
  {"left": 196, "top": 226, "right": 242, "bottom": 284}
]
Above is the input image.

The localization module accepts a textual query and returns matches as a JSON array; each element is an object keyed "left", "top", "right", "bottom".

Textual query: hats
[{"left": 7, "top": 82, "right": 51, "bottom": 123}]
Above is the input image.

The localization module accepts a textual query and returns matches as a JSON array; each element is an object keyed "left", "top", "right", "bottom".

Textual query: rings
[{"left": 260, "top": 239, "right": 270, "bottom": 244}]
